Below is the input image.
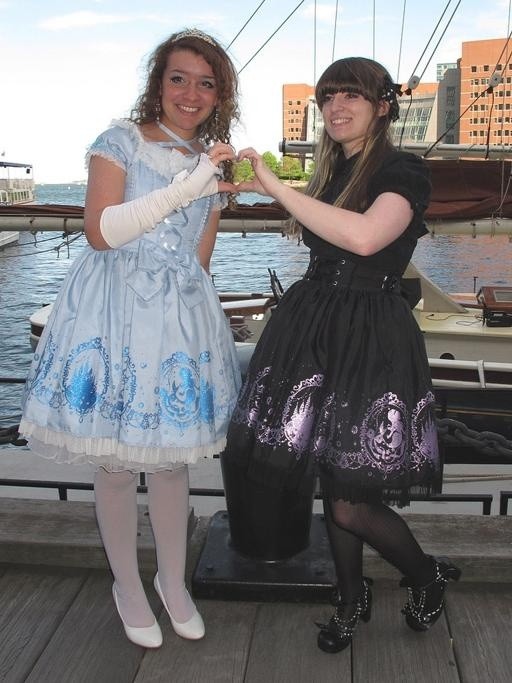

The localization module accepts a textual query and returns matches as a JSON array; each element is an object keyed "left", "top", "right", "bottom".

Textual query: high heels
[
  {"left": 153, "top": 572, "right": 206, "bottom": 640},
  {"left": 111, "top": 579, "right": 164, "bottom": 648},
  {"left": 316, "top": 575, "right": 373, "bottom": 654},
  {"left": 400, "top": 557, "right": 462, "bottom": 633}
]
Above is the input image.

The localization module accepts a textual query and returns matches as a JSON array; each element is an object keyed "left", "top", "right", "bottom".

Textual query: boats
[{"left": 29, "top": 259, "right": 511, "bottom": 464}]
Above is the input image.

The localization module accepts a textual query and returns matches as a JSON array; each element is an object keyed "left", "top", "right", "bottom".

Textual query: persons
[
  {"left": 13, "top": 25, "right": 239, "bottom": 649},
  {"left": 227, "top": 54, "right": 462, "bottom": 653}
]
